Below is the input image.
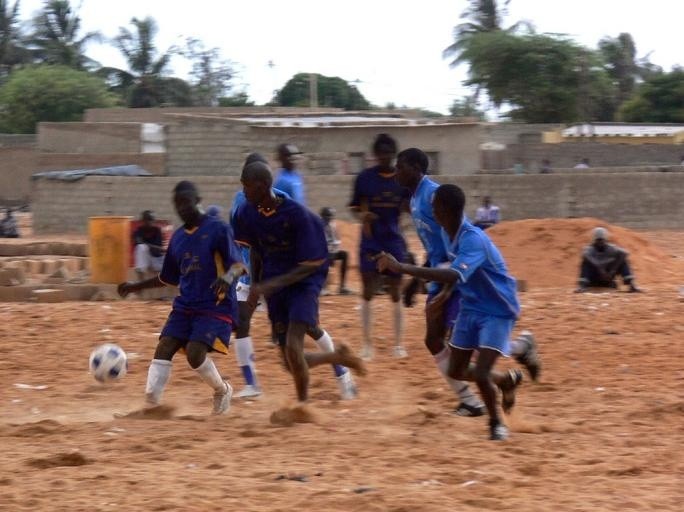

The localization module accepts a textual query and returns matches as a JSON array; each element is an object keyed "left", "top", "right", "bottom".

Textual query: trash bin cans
[{"left": 90, "top": 216, "right": 131, "bottom": 280}]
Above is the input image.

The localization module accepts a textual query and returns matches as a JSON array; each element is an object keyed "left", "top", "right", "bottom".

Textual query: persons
[
  {"left": 204, "top": 202, "right": 222, "bottom": 220},
  {"left": 470, "top": 196, "right": 502, "bottom": 231},
  {"left": 316, "top": 204, "right": 356, "bottom": 295},
  {"left": 345, "top": 131, "right": 412, "bottom": 362},
  {"left": 272, "top": 142, "right": 306, "bottom": 210},
  {"left": 512, "top": 157, "right": 525, "bottom": 175},
  {"left": 375, "top": 184, "right": 523, "bottom": 442},
  {"left": 225, "top": 221, "right": 262, "bottom": 399},
  {"left": 573, "top": 226, "right": 645, "bottom": 293},
  {"left": 130, "top": 208, "right": 167, "bottom": 281},
  {"left": 195, "top": 195, "right": 206, "bottom": 215},
  {"left": 539, "top": 158, "right": 556, "bottom": 174},
  {"left": 114, "top": 180, "right": 234, "bottom": 416},
  {"left": 392, "top": 148, "right": 540, "bottom": 417},
  {"left": 0, "top": 207, "right": 20, "bottom": 238},
  {"left": 573, "top": 158, "right": 590, "bottom": 168}
]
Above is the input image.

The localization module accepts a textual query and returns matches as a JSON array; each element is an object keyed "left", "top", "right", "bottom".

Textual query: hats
[{"left": 592, "top": 228, "right": 607, "bottom": 240}]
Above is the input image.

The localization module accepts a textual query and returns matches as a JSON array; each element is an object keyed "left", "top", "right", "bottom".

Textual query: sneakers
[
  {"left": 339, "top": 347, "right": 408, "bottom": 400},
  {"left": 213, "top": 382, "right": 260, "bottom": 413},
  {"left": 320, "top": 288, "right": 354, "bottom": 297},
  {"left": 454, "top": 332, "right": 539, "bottom": 440}
]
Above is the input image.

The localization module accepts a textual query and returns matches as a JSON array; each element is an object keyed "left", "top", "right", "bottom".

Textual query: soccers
[{"left": 90, "top": 343, "right": 128, "bottom": 384}]
